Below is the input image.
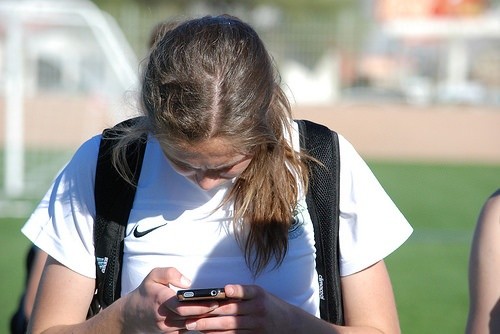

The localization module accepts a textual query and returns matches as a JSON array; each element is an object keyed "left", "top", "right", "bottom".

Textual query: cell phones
[{"left": 176, "top": 288, "right": 230, "bottom": 302}]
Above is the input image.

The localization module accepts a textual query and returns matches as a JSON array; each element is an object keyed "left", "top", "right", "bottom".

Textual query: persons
[
  {"left": 19, "top": 15, "right": 414, "bottom": 334},
  {"left": 466, "top": 186, "right": 499, "bottom": 334}
]
